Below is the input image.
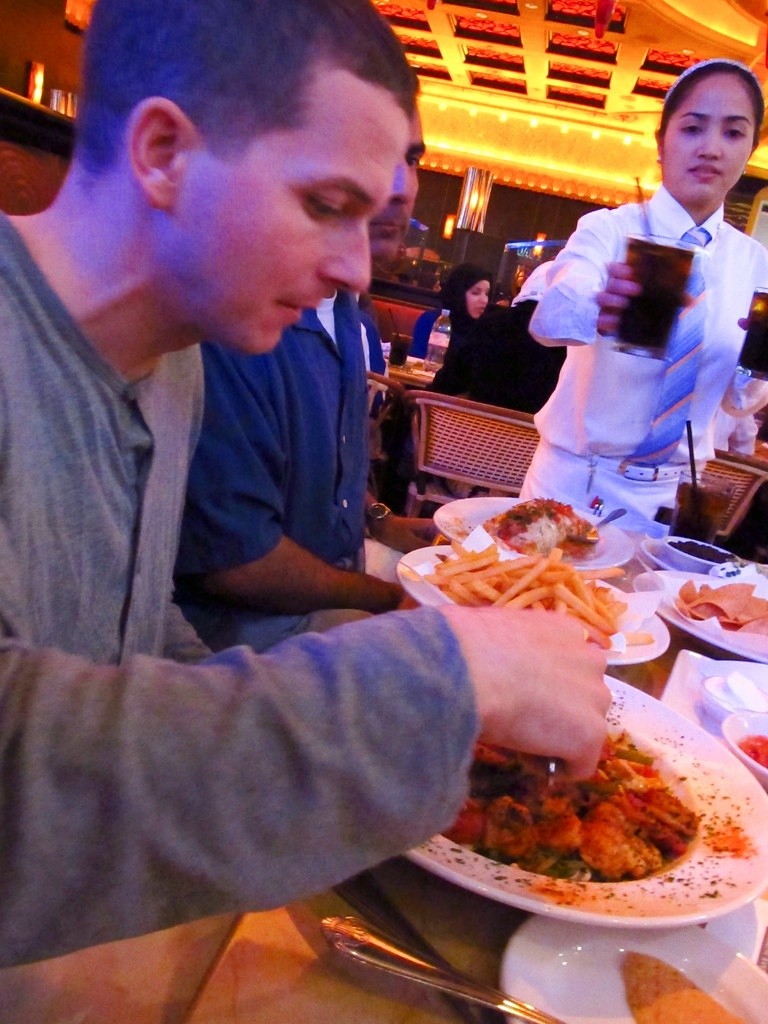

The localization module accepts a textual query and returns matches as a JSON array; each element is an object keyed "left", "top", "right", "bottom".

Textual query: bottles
[{"left": 423, "top": 308, "right": 451, "bottom": 373}]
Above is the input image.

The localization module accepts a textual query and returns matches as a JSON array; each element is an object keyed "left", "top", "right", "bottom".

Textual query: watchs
[{"left": 367, "top": 502, "right": 391, "bottom": 528}]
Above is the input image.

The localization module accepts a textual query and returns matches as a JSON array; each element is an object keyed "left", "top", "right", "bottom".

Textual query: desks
[
  {"left": 386, "top": 364, "right": 436, "bottom": 391},
  {"left": 752, "top": 438, "right": 768, "bottom": 465}
]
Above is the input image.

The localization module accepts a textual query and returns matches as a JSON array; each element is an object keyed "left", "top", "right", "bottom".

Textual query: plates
[
  {"left": 641, "top": 537, "right": 680, "bottom": 572},
  {"left": 396, "top": 544, "right": 671, "bottom": 665},
  {"left": 434, "top": 497, "right": 637, "bottom": 571},
  {"left": 632, "top": 570, "right": 768, "bottom": 663},
  {"left": 399, "top": 650, "right": 768, "bottom": 1024}
]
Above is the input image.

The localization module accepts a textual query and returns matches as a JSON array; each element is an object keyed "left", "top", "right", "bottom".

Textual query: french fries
[{"left": 423, "top": 541, "right": 654, "bottom": 649}]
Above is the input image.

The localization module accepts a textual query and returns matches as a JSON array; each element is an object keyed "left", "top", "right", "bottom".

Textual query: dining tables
[{"left": 181, "top": 532, "right": 750, "bottom": 1024}]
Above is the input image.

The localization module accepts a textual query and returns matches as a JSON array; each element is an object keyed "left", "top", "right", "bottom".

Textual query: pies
[{"left": 623, "top": 951, "right": 747, "bottom": 1024}]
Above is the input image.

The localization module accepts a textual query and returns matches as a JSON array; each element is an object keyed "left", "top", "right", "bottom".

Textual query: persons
[
  {"left": 0, "top": 0, "right": 612, "bottom": 960},
  {"left": 519, "top": 58, "right": 768, "bottom": 527},
  {"left": 361, "top": 259, "right": 757, "bottom": 516},
  {"left": 174, "top": 98, "right": 435, "bottom": 658}
]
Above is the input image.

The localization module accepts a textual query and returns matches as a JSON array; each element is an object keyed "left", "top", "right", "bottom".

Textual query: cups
[
  {"left": 50, "top": 89, "right": 77, "bottom": 119},
  {"left": 735, "top": 287, "right": 768, "bottom": 384},
  {"left": 613, "top": 231, "right": 698, "bottom": 361},
  {"left": 667, "top": 470, "right": 737, "bottom": 545},
  {"left": 389, "top": 332, "right": 415, "bottom": 369}
]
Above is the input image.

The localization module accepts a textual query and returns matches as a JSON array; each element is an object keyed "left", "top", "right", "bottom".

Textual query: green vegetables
[{"left": 465, "top": 744, "right": 655, "bottom": 879}]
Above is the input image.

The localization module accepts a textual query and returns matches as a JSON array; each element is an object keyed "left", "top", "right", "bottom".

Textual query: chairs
[
  {"left": 700, "top": 446, "right": 768, "bottom": 539},
  {"left": 363, "top": 371, "right": 539, "bottom": 521}
]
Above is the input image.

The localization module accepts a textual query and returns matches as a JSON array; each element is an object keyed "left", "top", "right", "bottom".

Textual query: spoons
[{"left": 567, "top": 508, "right": 626, "bottom": 543}]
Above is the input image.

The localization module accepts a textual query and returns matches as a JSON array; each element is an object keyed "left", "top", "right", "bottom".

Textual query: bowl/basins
[
  {"left": 693, "top": 661, "right": 768, "bottom": 722},
  {"left": 720, "top": 712, "right": 768, "bottom": 791},
  {"left": 662, "top": 536, "right": 741, "bottom": 574}
]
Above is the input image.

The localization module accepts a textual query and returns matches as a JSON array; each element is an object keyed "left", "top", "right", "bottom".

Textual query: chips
[{"left": 676, "top": 579, "right": 768, "bottom": 628}]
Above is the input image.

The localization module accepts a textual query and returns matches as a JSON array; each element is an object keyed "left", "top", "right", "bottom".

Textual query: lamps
[{"left": 453, "top": 166, "right": 493, "bottom": 236}]
